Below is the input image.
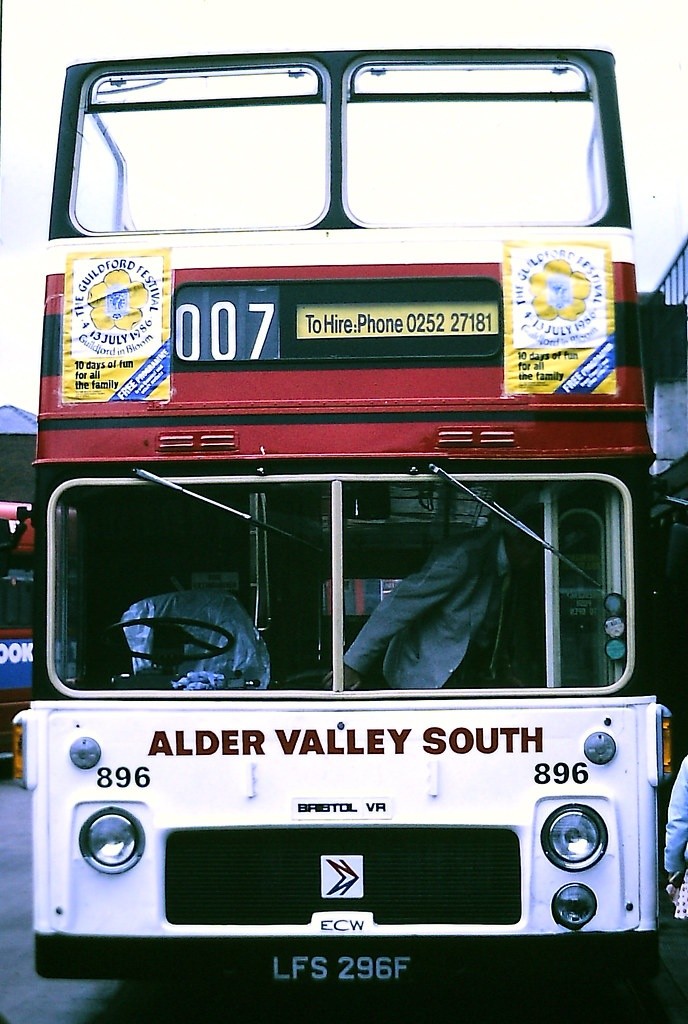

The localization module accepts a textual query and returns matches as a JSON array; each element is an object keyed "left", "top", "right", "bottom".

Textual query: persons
[
  {"left": 663, "top": 752, "right": 688, "bottom": 920},
  {"left": 327, "top": 507, "right": 517, "bottom": 697}
]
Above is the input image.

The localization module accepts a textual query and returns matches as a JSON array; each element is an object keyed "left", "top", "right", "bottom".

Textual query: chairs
[{"left": 122, "top": 588, "right": 269, "bottom": 689}]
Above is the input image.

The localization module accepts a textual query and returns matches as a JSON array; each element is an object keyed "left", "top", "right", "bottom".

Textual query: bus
[{"left": 0, "top": 45, "right": 675, "bottom": 990}]
